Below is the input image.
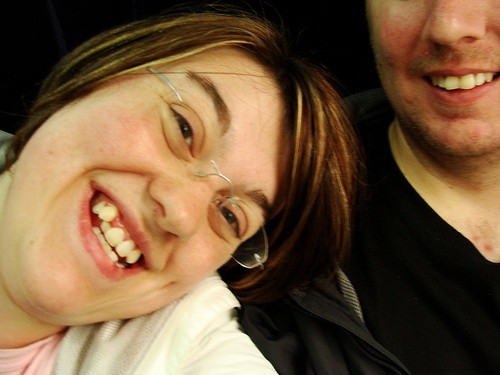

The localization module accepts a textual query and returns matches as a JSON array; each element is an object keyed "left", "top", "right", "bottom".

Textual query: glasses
[{"left": 147, "top": 66, "right": 269, "bottom": 268}]
